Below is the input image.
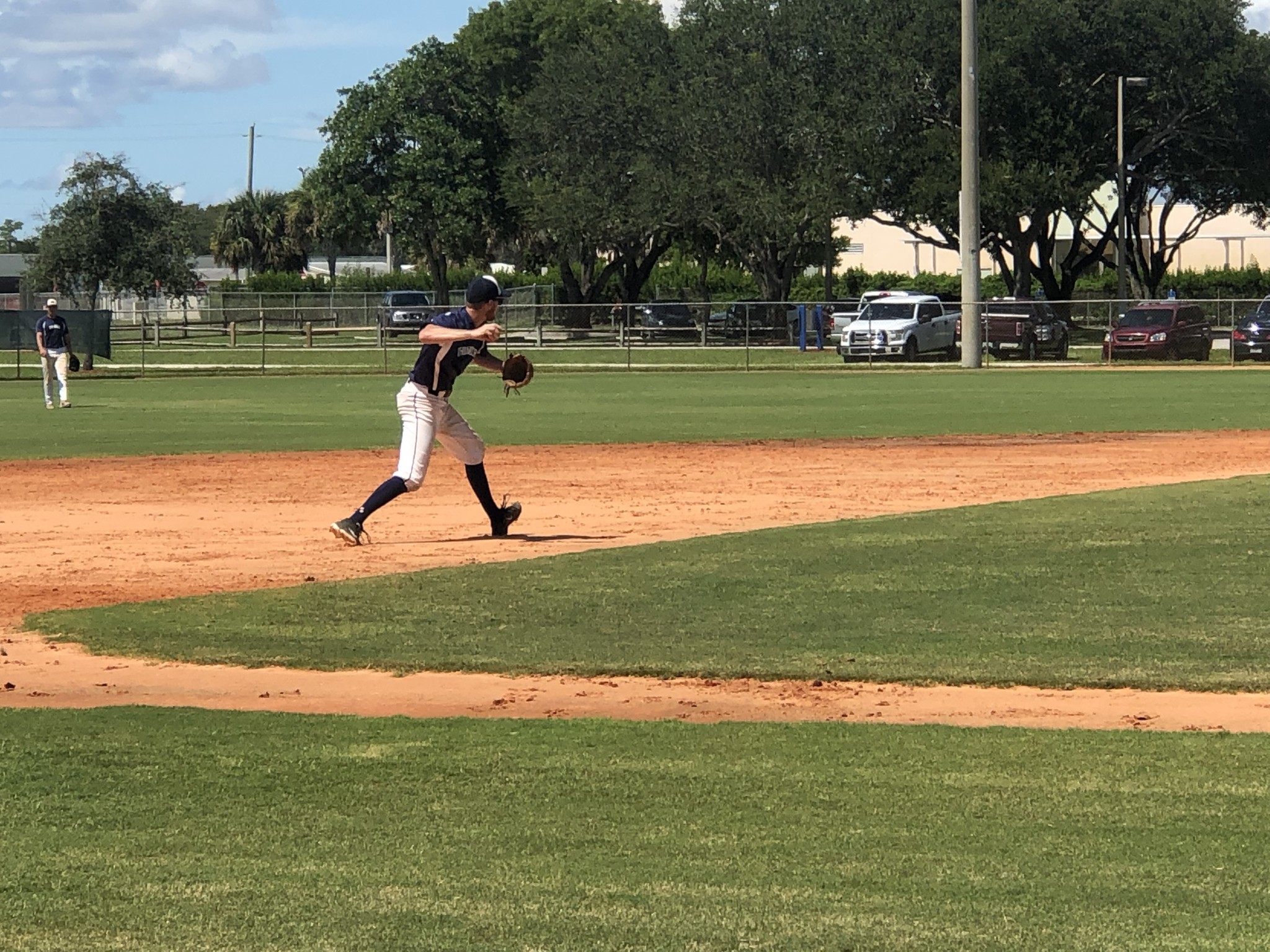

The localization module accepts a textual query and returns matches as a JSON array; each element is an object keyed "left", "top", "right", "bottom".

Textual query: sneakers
[
  {"left": 330, "top": 519, "right": 364, "bottom": 546},
  {"left": 492, "top": 501, "right": 521, "bottom": 535}
]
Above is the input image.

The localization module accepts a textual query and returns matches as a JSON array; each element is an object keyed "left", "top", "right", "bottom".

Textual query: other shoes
[
  {"left": 59, "top": 400, "right": 72, "bottom": 408},
  {"left": 47, "top": 403, "right": 55, "bottom": 409}
]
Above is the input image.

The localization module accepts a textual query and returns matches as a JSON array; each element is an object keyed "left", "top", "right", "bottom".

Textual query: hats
[
  {"left": 466, "top": 274, "right": 512, "bottom": 302},
  {"left": 46, "top": 297, "right": 59, "bottom": 307}
]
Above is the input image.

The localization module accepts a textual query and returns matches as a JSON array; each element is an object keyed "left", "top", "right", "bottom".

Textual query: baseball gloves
[
  {"left": 496, "top": 352, "right": 534, "bottom": 398},
  {"left": 68, "top": 354, "right": 80, "bottom": 372}
]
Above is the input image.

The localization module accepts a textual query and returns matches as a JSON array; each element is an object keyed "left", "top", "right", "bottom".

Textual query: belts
[{"left": 428, "top": 389, "right": 452, "bottom": 398}]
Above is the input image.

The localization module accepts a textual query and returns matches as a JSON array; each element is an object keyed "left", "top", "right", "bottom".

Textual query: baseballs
[{"left": 493, "top": 330, "right": 500, "bottom": 340}]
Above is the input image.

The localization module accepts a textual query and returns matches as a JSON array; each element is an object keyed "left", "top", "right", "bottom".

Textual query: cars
[
  {"left": 1230, "top": 313, "right": 1270, "bottom": 362},
  {"left": 708, "top": 301, "right": 810, "bottom": 338},
  {"left": 641, "top": 300, "right": 698, "bottom": 336},
  {"left": 378, "top": 291, "right": 436, "bottom": 338},
  {"left": 1102, "top": 303, "right": 1214, "bottom": 361}
]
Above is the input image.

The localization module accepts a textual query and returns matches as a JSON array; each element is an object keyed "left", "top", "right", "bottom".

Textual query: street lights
[{"left": 1117, "top": 75, "right": 1148, "bottom": 310}]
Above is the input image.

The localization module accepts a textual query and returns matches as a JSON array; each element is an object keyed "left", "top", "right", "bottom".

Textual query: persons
[
  {"left": 35, "top": 298, "right": 80, "bottom": 410},
  {"left": 329, "top": 274, "right": 534, "bottom": 547}
]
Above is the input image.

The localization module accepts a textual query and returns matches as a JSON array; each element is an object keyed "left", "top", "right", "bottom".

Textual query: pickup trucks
[
  {"left": 841, "top": 296, "right": 962, "bottom": 362},
  {"left": 829, "top": 290, "right": 925, "bottom": 355},
  {"left": 954, "top": 296, "right": 1069, "bottom": 361}
]
